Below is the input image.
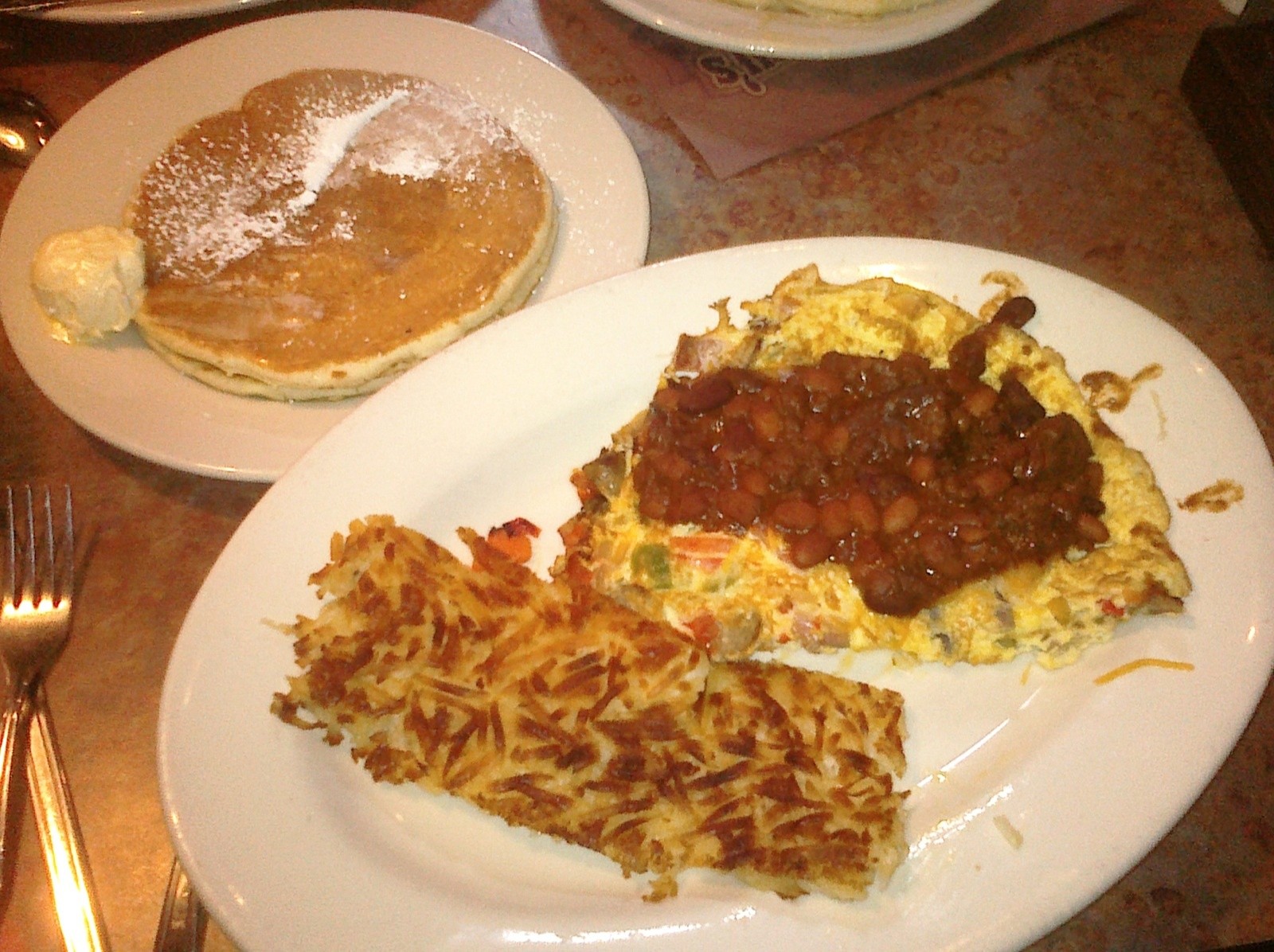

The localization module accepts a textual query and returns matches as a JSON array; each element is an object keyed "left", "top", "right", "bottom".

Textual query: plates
[
  {"left": 150, "top": 233, "right": 1273, "bottom": 952},
  {"left": 604, "top": 2, "right": 998, "bottom": 62},
  {"left": 1, "top": 10, "right": 649, "bottom": 481}
]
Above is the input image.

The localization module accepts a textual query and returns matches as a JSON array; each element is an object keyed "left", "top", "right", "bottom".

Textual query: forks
[{"left": 0, "top": 476, "right": 75, "bottom": 890}]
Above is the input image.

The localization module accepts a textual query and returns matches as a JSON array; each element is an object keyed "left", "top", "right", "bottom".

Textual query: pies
[
  {"left": 596, "top": 258, "right": 1195, "bottom": 661},
  {"left": 121, "top": 50, "right": 559, "bottom": 410},
  {"left": 250, "top": 507, "right": 916, "bottom": 909}
]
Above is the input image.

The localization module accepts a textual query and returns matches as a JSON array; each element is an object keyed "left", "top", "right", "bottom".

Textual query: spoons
[{"left": 2, "top": 83, "right": 57, "bottom": 167}]
